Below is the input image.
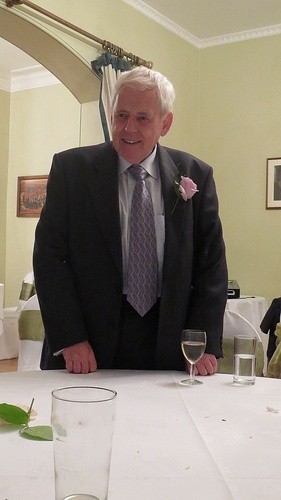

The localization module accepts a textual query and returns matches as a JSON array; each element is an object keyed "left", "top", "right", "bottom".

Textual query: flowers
[{"left": 168, "top": 175, "right": 199, "bottom": 213}]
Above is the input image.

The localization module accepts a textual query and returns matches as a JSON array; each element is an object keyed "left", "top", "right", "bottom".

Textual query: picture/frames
[
  {"left": 17, "top": 175, "right": 48, "bottom": 218},
  {"left": 265, "top": 157, "right": 281, "bottom": 209}
]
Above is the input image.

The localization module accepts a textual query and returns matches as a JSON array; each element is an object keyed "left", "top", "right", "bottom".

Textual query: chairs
[
  {"left": 219, "top": 311, "right": 271, "bottom": 376},
  {"left": 0, "top": 271, "right": 45, "bottom": 371}
]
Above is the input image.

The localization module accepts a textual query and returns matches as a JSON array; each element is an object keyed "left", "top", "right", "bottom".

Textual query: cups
[
  {"left": 233, "top": 354, "right": 256, "bottom": 386},
  {"left": 234, "top": 335, "right": 258, "bottom": 354},
  {"left": 51, "top": 386, "right": 117, "bottom": 500}
]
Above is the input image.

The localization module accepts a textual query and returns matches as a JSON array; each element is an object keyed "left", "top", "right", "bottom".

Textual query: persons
[{"left": 33, "top": 65, "right": 228, "bottom": 376}]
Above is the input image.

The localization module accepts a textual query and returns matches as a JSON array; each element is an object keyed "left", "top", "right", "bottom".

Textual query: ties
[{"left": 126, "top": 164, "right": 159, "bottom": 316}]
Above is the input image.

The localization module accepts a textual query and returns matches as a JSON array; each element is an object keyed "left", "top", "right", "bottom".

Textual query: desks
[
  {"left": 220, "top": 295, "right": 270, "bottom": 376},
  {"left": 0, "top": 369, "right": 281, "bottom": 500}
]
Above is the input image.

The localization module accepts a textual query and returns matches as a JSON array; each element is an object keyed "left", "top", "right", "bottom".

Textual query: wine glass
[{"left": 179, "top": 329, "right": 206, "bottom": 386}]
